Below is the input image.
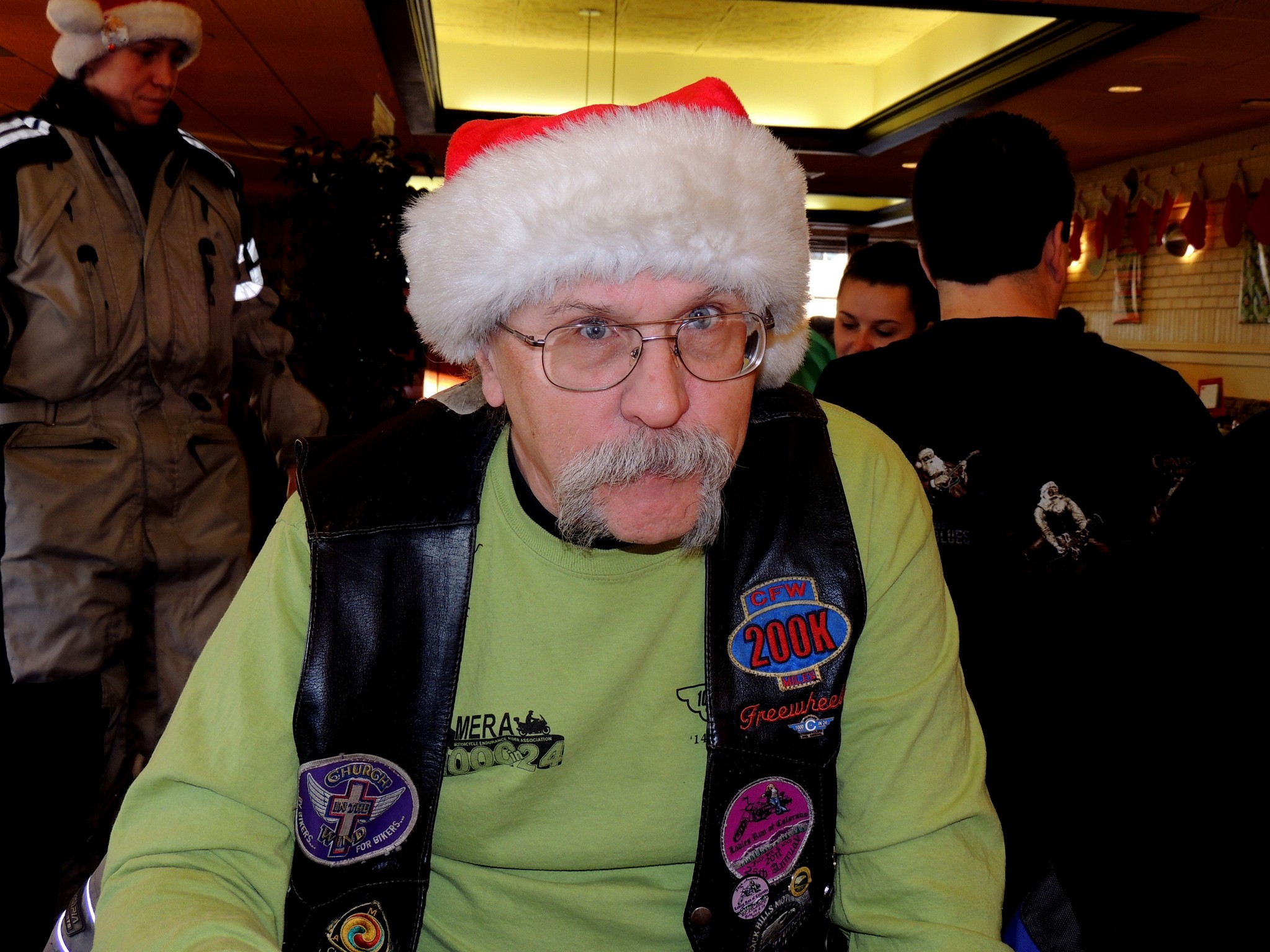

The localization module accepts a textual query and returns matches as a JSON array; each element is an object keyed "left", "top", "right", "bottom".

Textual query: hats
[
  {"left": 44, "top": 0, "right": 205, "bottom": 79},
  {"left": 396, "top": 79, "right": 812, "bottom": 388}
]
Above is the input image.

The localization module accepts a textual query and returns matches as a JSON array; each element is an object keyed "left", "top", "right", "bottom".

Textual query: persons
[
  {"left": 832, "top": 239, "right": 940, "bottom": 360},
  {"left": 86, "top": 74, "right": 1011, "bottom": 952},
  {"left": 1, "top": 0, "right": 253, "bottom": 950},
  {"left": 812, "top": 105, "right": 1230, "bottom": 952},
  {"left": 1033, "top": 480, "right": 1110, "bottom": 576},
  {"left": 912, "top": 448, "right": 971, "bottom": 508},
  {"left": 786, "top": 316, "right": 838, "bottom": 395}
]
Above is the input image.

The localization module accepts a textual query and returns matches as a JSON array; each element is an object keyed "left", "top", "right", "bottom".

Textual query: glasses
[{"left": 488, "top": 310, "right": 775, "bottom": 393}]
